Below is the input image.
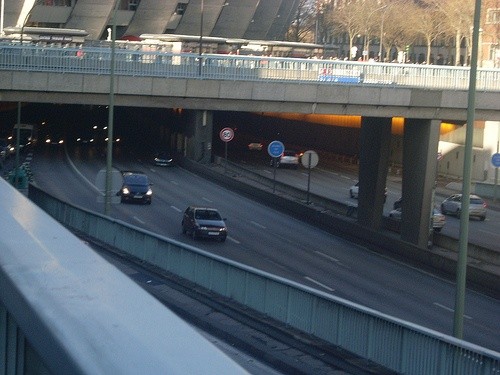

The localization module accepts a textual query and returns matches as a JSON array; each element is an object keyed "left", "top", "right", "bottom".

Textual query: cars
[
  {"left": 389, "top": 207, "right": 446, "bottom": 232},
  {"left": 182, "top": 206, "right": 228, "bottom": 242},
  {"left": 0, "top": 119, "right": 121, "bottom": 160},
  {"left": 349, "top": 182, "right": 388, "bottom": 201},
  {"left": 441, "top": 194, "right": 487, "bottom": 220},
  {"left": 234, "top": 126, "right": 305, "bottom": 169},
  {"left": 120, "top": 174, "right": 154, "bottom": 204},
  {"left": 154, "top": 151, "right": 174, "bottom": 167}
]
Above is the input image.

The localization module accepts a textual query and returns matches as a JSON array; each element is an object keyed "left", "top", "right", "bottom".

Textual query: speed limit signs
[{"left": 220, "top": 128, "right": 234, "bottom": 142}]
